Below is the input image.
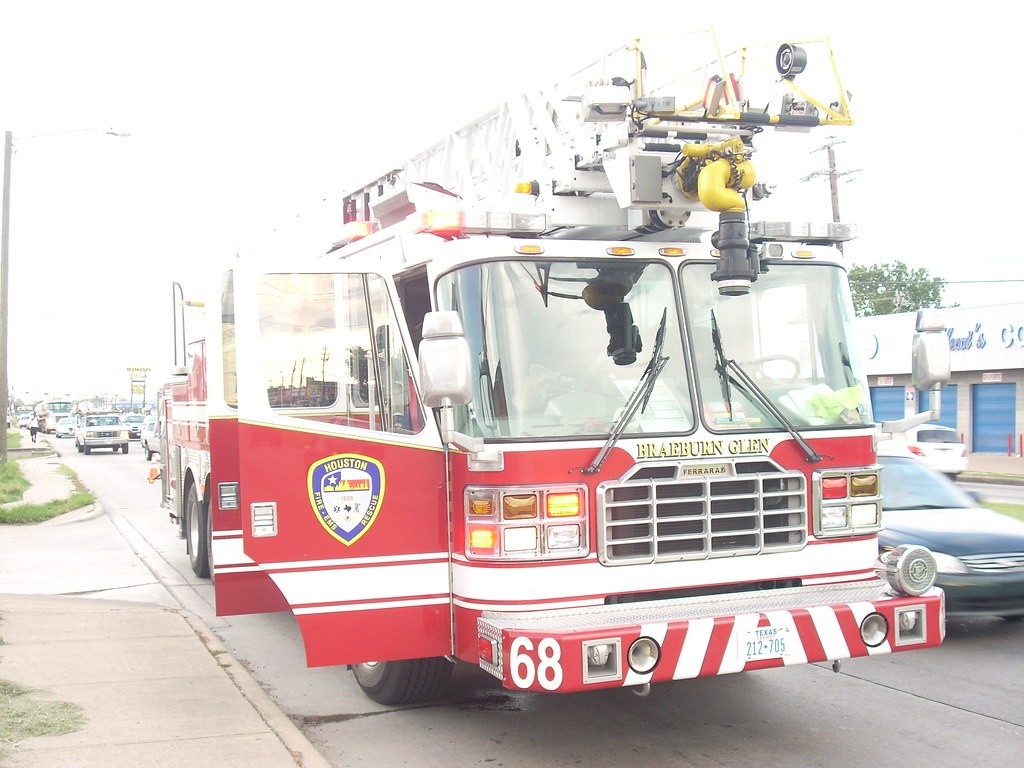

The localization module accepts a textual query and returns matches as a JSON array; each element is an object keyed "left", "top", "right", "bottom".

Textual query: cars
[
  {"left": 6, "top": 398, "right": 160, "bottom": 461},
  {"left": 876, "top": 452, "right": 1024, "bottom": 624}
]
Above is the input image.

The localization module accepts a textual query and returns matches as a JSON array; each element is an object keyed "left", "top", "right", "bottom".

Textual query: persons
[
  {"left": 28, "top": 412, "right": 39, "bottom": 443},
  {"left": 7, "top": 413, "right": 12, "bottom": 428}
]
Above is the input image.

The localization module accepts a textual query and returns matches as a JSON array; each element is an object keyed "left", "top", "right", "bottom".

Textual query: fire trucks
[{"left": 158, "top": 32, "right": 950, "bottom": 707}]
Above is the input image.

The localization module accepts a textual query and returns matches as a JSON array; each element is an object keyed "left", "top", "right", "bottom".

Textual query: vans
[{"left": 873, "top": 419, "right": 968, "bottom": 483}]
